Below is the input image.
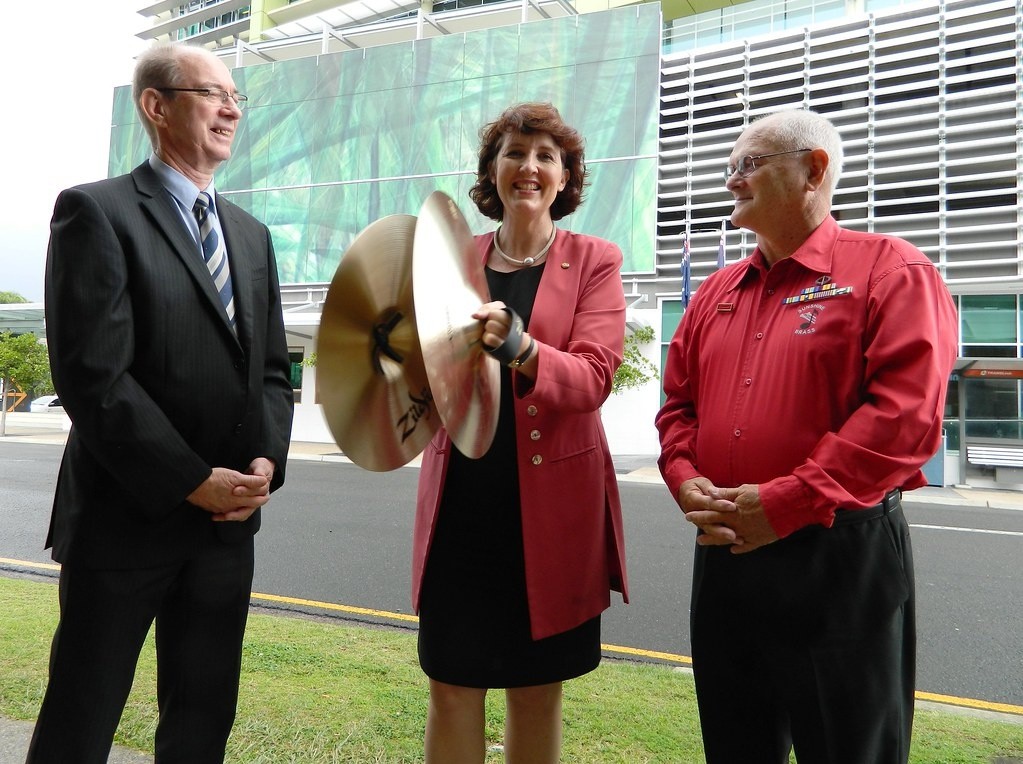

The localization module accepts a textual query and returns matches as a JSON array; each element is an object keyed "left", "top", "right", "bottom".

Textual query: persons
[
  {"left": 26, "top": 39, "right": 295, "bottom": 764},
  {"left": 656, "top": 110, "right": 960, "bottom": 764},
  {"left": 412, "top": 101, "right": 629, "bottom": 764}
]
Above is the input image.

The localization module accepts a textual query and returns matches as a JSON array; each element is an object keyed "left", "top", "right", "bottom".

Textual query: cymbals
[
  {"left": 315, "top": 215, "right": 442, "bottom": 473},
  {"left": 413, "top": 191, "right": 499, "bottom": 459}
]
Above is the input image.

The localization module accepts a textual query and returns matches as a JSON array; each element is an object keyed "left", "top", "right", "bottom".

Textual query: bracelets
[{"left": 508, "top": 336, "right": 534, "bottom": 368}]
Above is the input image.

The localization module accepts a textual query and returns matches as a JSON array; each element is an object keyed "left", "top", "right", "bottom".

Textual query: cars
[{"left": 30, "top": 396, "right": 66, "bottom": 413}]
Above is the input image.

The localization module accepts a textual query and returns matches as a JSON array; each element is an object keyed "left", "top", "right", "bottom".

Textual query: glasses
[
  {"left": 158, "top": 86, "right": 246, "bottom": 109},
  {"left": 724, "top": 148, "right": 814, "bottom": 178}
]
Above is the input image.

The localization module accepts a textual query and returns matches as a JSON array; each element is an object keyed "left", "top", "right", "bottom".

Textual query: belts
[{"left": 801, "top": 488, "right": 900, "bottom": 526}]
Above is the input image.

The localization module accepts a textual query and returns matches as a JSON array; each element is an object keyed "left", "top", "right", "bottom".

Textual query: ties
[{"left": 193, "top": 192, "right": 239, "bottom": 341}]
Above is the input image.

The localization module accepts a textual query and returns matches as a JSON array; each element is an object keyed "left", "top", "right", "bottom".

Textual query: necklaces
[{"left": 494, "top": 220, "right": 556, "bottom": 267}]
